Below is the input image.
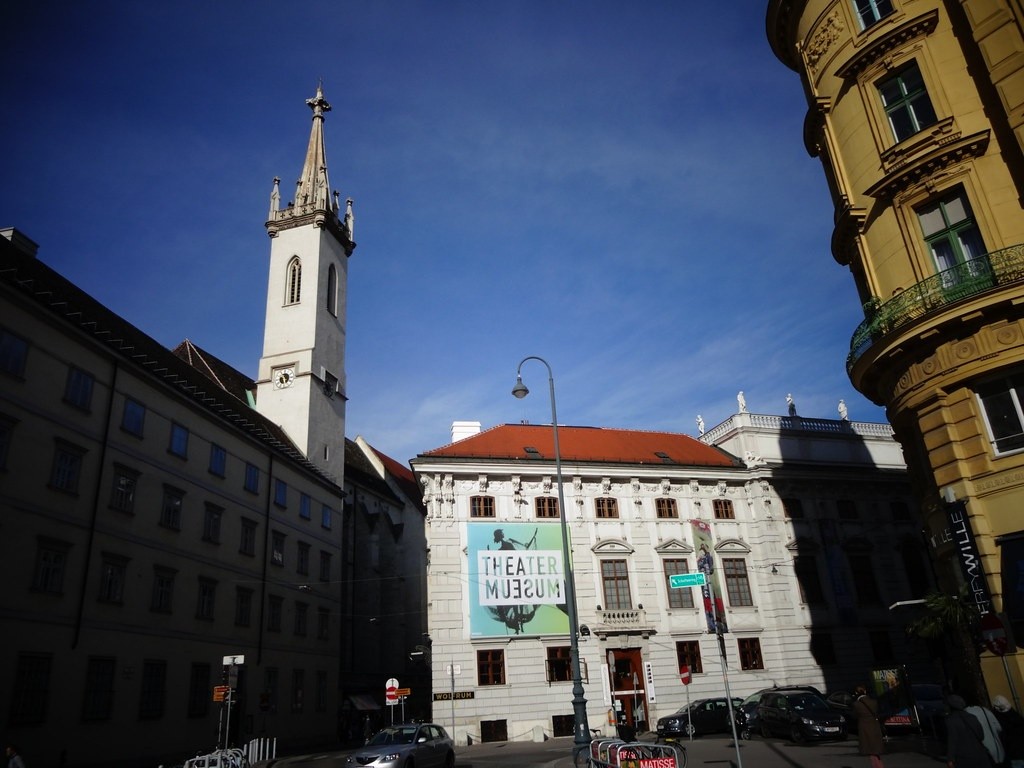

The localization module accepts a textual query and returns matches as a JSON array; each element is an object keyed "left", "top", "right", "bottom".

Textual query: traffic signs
[{"left": 670, "top": 573, "right": 706, "bottom": 588}]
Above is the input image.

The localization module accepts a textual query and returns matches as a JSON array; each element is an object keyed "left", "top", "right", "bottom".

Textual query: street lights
[{"left": 510, "top": 355, "right": 594, "bottom": 768}]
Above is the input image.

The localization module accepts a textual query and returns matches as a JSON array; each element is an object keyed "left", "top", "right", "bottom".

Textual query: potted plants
[{"left": 906, "top": 589, "right": 985, "bottom": 654}]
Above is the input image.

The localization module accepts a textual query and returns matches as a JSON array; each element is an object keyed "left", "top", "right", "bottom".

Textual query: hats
[
  {"left": 948, "top": 695, "right": 966, "bottom": 711},
  {"left": 993, "top": 695, "right": 1011, "bottom": 712}
]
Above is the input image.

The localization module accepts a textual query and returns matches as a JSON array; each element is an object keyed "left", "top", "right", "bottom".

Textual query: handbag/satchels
[{"left": 881, "top": 722, "right": 887, "bottom": 737}]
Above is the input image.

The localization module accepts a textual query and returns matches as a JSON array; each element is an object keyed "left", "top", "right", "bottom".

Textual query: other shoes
[{"left": 875, "top": 754, "right": 880, "bottom": 760}]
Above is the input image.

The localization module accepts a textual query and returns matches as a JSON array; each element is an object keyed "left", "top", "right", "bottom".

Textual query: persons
[
  {"left": 944, "top": 695, "right": 993, "bottom": 768},
  {"left": 338, "top": 712, "right": 385, "bottom": 745},
  {"left": 962, "top": 693, "right": 1024, "bottom": 768},
  {"left": 3, "top": 745, "right": 26, "bottom": 768},
  {"left": 853, "top": 687, "right": 885, "bottom": 768}
]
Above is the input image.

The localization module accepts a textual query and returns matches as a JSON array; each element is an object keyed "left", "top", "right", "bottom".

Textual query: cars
[
  {"left": 341, "top": 722, "right": 455, "bottom": 768},
  {"left": 734, "top": 684, "right": 857, "bottom": 743}
]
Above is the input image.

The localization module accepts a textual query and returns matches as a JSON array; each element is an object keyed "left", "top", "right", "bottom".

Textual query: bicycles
[{"left": 574, "top": 721, "right": 688, "bottom": 768}]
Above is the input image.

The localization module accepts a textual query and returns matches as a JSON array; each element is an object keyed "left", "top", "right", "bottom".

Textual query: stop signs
[
  {"left": 387, "top": 687, "right": 398, "bottom": 699},
  {"left": 980, "top": 614, "right": 1008, "bottom": 656},
  {"left": 679, "top": 665, "right": 690, "bottom": 685}
]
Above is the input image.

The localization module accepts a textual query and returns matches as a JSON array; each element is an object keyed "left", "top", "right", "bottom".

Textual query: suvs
[{"left": 656, "top": 696, "right": 745, "bottom": 738}]
